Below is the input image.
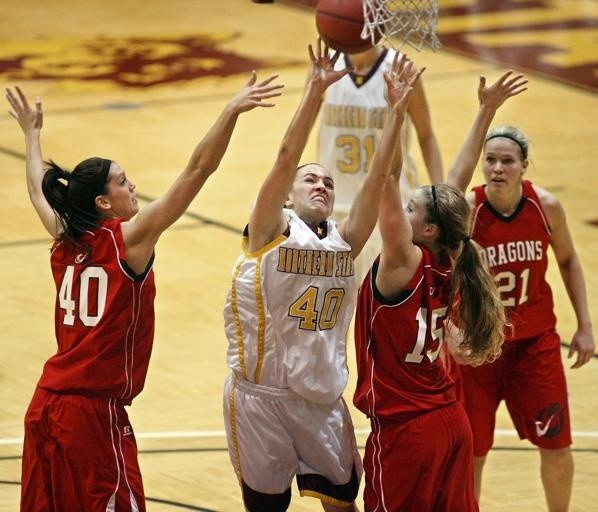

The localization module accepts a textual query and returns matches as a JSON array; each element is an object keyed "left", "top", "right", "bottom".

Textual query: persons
[
  {"left": 5, "top": 69, "right": 285, "bottom": 511},
  {"left": 372, "top": 49, "right": 529, "bottom": 512},
  {"left": 312, "top": 43, "right": 445, "bottom": 289},
  {"left": 457, "top": 121, "right": 596, "bottom": 512},
  {"left": 222, "top": 39, "right": 426, "bottom": 511}
]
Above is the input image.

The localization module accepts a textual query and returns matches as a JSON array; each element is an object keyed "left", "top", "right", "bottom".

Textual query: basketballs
[{"left": 316, "top": 0, "right": 388, "bottom": 54}]
